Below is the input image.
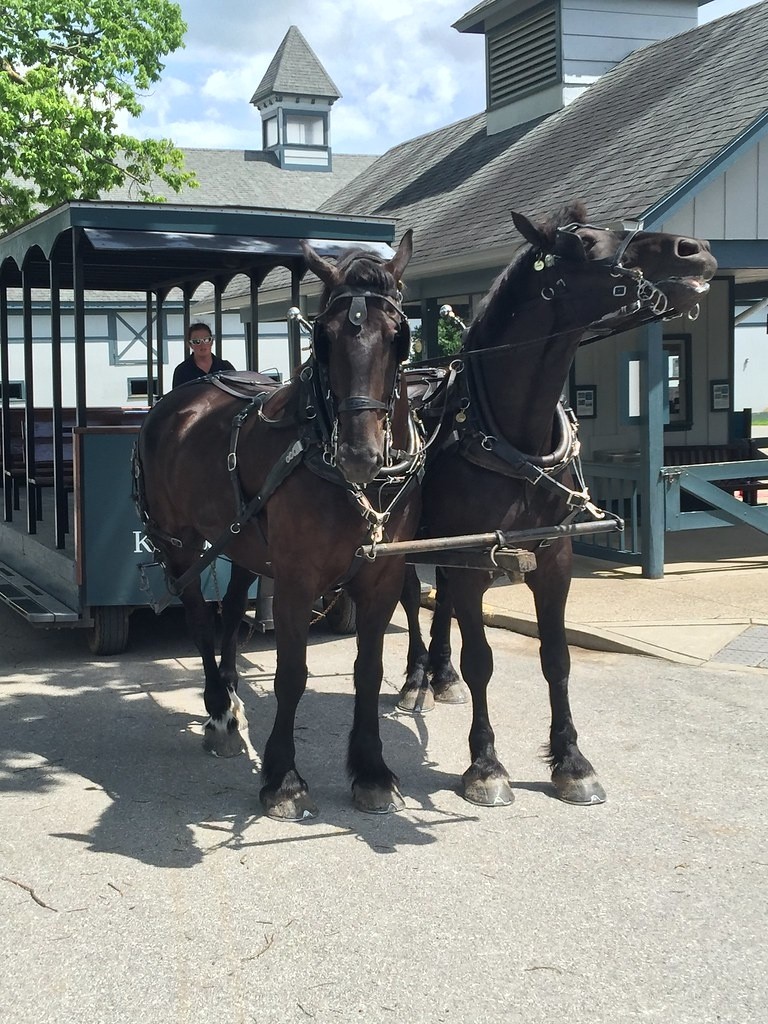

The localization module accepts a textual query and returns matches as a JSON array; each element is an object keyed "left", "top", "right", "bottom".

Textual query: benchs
[
  {"left": 665, "top": 444, "right": 768, "bottom": 506},
  {"left": 0, "top": 407, "right": 152, "bottom": 549}
]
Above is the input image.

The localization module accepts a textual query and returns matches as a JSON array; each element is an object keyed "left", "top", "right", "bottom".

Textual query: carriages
[{"left": 1, "top": 201, "right": 718, "bottom": 823}]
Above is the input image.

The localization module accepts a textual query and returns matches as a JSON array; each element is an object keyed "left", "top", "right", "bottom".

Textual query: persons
[{"left": 172, "top": 324, "right": 236, "bottom": 389}]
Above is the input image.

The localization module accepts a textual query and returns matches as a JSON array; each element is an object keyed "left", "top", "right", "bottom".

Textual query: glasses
[{"left": 188, "top": 337, "right": 214, "bottom": 345}]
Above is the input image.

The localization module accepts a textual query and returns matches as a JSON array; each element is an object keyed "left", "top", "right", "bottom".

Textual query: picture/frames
[
  {"left": 710, "top": 379, "right": 733, "bottom": 412},
  {"left": 574, "top": 385, "right": 597, "bottom": 419}
]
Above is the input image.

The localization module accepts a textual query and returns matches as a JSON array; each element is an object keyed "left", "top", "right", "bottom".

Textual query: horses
[{"left": 129, "top": 199, "right": 721, "bottom": 823}]
